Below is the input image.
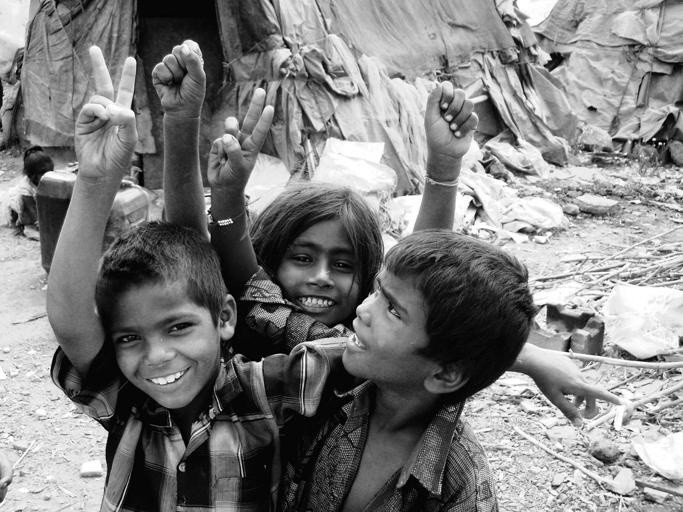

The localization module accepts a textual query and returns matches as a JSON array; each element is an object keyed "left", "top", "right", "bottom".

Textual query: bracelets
[
  {"left": 209, "top": 223, "right": 250, "bottom": 247},
  {"left": 208, "top": 194, "right": 249, "bottom": 227},
  {"left": 422, "top": 173, "right": 459, "bottom": 190}
]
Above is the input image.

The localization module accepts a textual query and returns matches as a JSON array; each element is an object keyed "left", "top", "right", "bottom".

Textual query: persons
[
  {"left": 44, "top": 46, "right": 626, "bottom": 512},
  {"left": 6, "top": 146, "right": 52, "bottom": 241},
  {"left": 151, "top": 38, "right": 480, "bottom": 512},
  {"left": 205, "top": 87, "right": 535, "bottom": 512}
]
